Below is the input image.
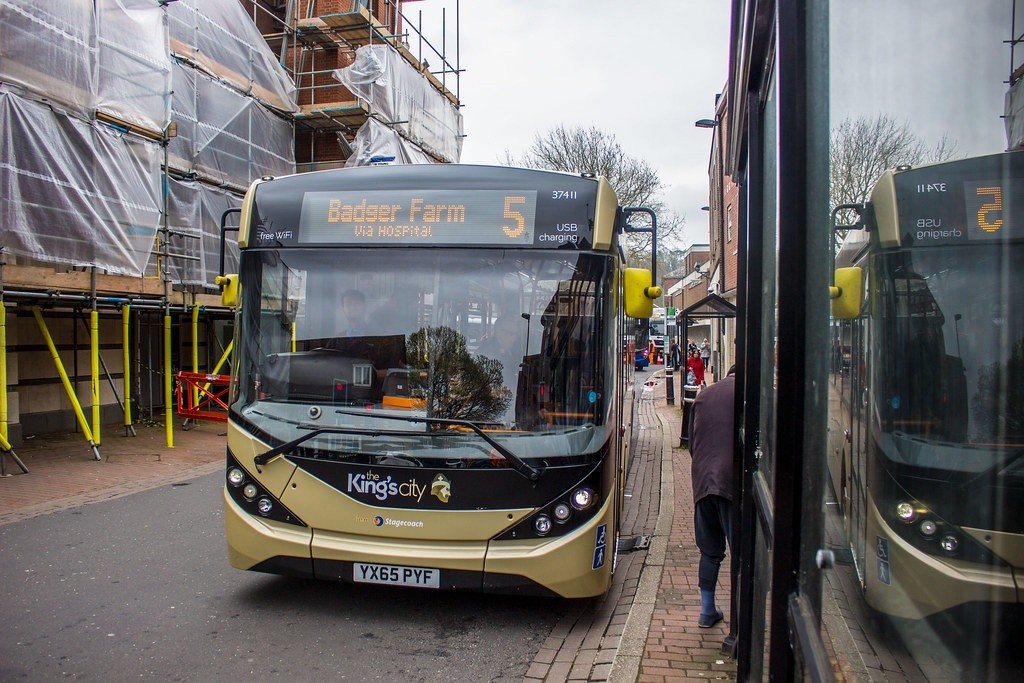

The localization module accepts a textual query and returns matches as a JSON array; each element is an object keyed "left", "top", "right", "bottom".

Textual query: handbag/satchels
[{"left": 687, "top": 370, "right": 697, "bottom": 386}]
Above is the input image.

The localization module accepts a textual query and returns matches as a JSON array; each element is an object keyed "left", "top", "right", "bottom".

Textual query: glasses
[{"left": 500, "top": 328, "right": 519, "bottom": 336}]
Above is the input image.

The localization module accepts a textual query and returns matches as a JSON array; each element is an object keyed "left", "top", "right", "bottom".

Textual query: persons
[
  {"left": 687, "top": 349, "right": 707, "bottom": 387},
  {"left": 648, "top": 340, "right": 656, "bottom": 366},
  {"left": 669, "top": 338, "right": 681, "bottom": 372},
  {"left": 701, "top": 338, "right": 711, "bottom": 369},
  {"left": 687, "top": 340, "right": 701, "bottom": 359},
  {"left": 326, "top": 289, "right": 381, "bottom": 362},
  {"left": 690, "top": 364, "right": 742, "bottom": 636},
  {"left": 473, "top": 314, "right": 523, "bottom": 369}
]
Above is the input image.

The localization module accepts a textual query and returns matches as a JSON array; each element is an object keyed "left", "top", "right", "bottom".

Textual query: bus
[
  {"left": 213, "top": 161, "right": 664, "bottom": 607},
  {"left": 828, "top": 147, "right": 1024, "bottom": 644},
  {"left": 631, "top": 316, "right": 651, "bottom": 373}
]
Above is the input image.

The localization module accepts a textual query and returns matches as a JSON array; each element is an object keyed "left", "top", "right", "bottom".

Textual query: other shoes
[{"left": 698, "top": 607, "right": 724, "bottom": 627}]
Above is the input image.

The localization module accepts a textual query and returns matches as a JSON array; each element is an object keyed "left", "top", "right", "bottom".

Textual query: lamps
[
  {"left": 701, "top": 206, "right": 709, "bottom": 210},
  {"left": 695, "top": 265, "right": 710, "bottom": 279},
  {"left": 707, "top": 282, "right": 720, "bottom": 293},
  {"left": 695, "top": 119, "right": 716, "bottom": 128}
]
